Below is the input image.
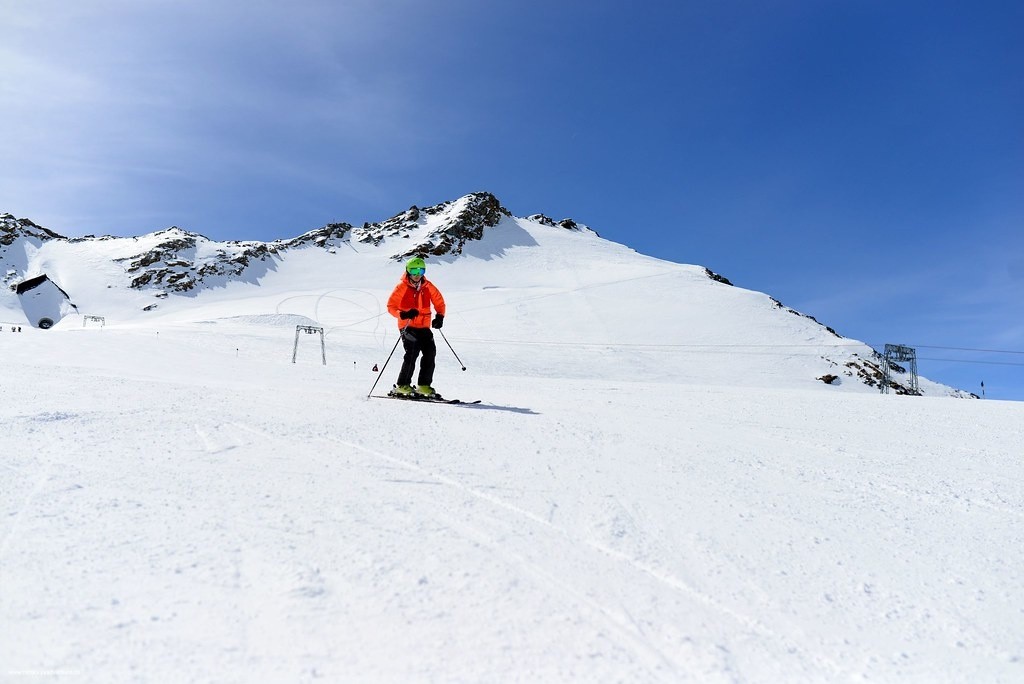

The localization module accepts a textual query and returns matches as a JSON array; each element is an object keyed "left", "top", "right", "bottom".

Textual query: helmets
[{"left": 406, "top": 257, "right": 426, "bottom": 276}]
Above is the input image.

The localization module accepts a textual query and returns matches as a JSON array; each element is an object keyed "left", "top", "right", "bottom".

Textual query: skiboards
[{"left": 372, "top": 393, "right": 481, "bottom": 406}]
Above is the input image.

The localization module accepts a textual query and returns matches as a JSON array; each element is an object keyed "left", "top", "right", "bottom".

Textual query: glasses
[{"left": 411, "top": 269, "right": 424, "bottom": 275}]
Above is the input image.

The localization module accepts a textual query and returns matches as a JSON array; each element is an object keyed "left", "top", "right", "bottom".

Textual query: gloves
[
  {"left": 432, "top": 313, "right": 444, "bottom": 329},
  {"left": 400, "top": 308, "right": 419, "bottom": 320}
]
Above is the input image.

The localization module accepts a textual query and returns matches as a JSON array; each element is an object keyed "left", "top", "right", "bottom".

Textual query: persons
[{"left": 386, "top": 257, "right": 445, "bottom": 399}]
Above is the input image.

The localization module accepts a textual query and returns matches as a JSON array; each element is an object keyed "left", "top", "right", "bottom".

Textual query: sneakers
[
  {"left": 395, "top": 384, "right": 415, "bottom": 394},
  {"left": 418, "top": 385, "right": 435, "bottom": 394}
]
